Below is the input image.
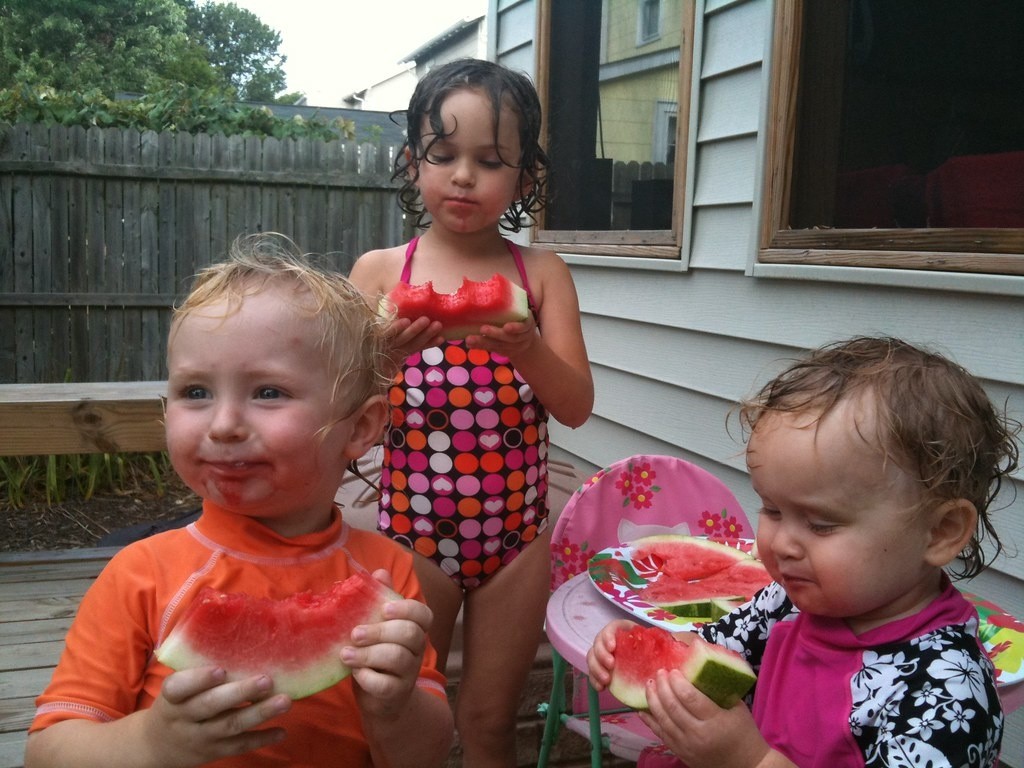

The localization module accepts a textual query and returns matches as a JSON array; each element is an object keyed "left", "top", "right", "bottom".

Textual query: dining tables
[{"left": 545, "top": 550, "right": 1024, "bottom": 768}]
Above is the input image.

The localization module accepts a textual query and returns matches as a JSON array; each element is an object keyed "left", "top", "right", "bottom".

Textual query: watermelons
[
  {"left": 632, "top": 535, "right": 775, "bottom": 623},
  {"left": 607, "top": 625, "right": 758, "bottom": 711},
  {"left": 154, "top": 573, "right": 406, "bottom": 701},
  {"left": 377, "top": 273, "right": 528, "bottom": 341}
]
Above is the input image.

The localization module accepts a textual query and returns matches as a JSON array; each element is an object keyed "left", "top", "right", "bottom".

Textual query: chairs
[{"left": 538, "top": 453, "right": 756, "bottom": 768}]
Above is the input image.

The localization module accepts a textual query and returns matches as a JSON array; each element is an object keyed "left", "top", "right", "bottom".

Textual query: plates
[
  {"left": 588, "top": 540, "right": 715, "bottom": 633},
  {"left": 957, "top": 581, "right": 1024, "bottom": 690}
]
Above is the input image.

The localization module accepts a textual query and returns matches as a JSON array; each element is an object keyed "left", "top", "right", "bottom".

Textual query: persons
[
  {"left": 586, "top": 336, "right": 1024, "bottom": 768},
  {"left": 345, "top": 58, "right": 596, "bottom": 768},
  {"left": 24, "top": 231, "right": 455, "bottom": 768}
]
